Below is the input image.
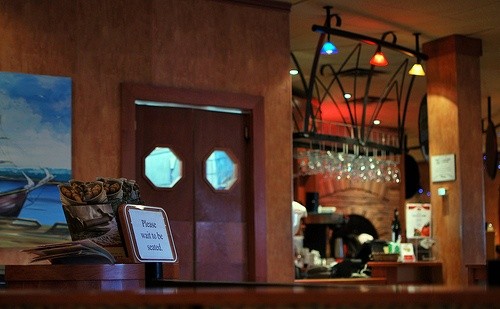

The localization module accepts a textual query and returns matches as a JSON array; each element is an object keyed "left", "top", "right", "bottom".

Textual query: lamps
[
  {"left": 371, "top": 32, "right": 390, "bottom": 68},
  {"left": 321, "top": 6, "right": 337, "bottom": 56},
  {"left": 410, "top": 31, "right": 426, "bottom": 76}
]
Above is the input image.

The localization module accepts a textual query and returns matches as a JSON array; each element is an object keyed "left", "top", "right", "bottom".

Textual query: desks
[
  {"left": 368, "top": 262, "right": 444, "bottom": 286},
  {"left": 466, "top": 264, "right": 487, "bottom": 288}
]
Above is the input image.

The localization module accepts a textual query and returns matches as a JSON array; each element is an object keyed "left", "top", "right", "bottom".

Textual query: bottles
[{"left": 391, "top": 208, "right": 402, "bottom": 243}]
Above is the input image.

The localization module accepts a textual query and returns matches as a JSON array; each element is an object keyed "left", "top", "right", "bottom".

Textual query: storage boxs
[{"left": 373, "top": 253, "right": 400, "bottom": 262}]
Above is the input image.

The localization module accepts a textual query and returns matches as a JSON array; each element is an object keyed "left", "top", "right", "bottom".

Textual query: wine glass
[{"left": 293, "top": 138, "right": 402, "bottom": 189}]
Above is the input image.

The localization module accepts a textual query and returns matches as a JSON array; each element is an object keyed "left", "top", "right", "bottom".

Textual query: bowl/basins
[{"left": 371, "top": 252, "right": 399, "bottom": 262}]
